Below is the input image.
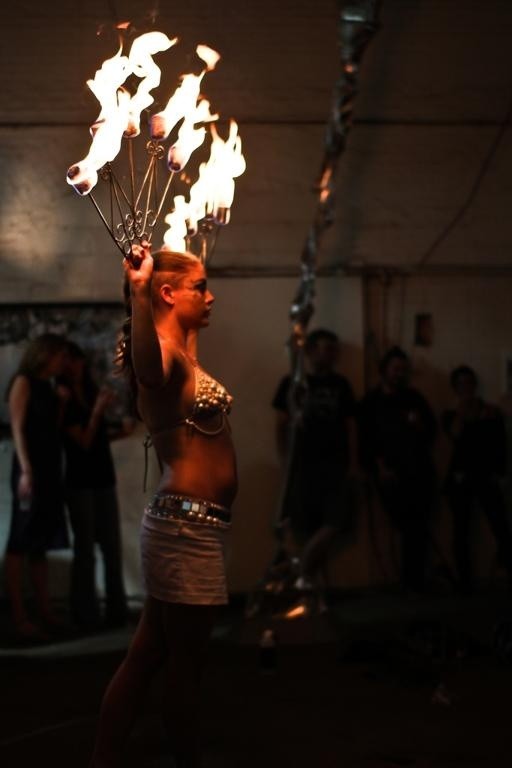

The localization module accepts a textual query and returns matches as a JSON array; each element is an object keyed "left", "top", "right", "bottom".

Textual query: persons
[{"left": 1, "top": 241, "right": 511, "bottom": 768}]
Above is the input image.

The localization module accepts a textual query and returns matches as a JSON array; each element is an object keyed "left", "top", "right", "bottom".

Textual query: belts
[{"left": 147, "top": 495, "right": 232, "bottom": 526}]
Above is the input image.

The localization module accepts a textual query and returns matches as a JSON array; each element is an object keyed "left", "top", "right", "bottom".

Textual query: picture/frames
[
  {"left": 501, "top": 351, "right": 512, "bottom": 394},
  {"left": 0, "top": 300, "right": 137, "bottom": 433}
]
